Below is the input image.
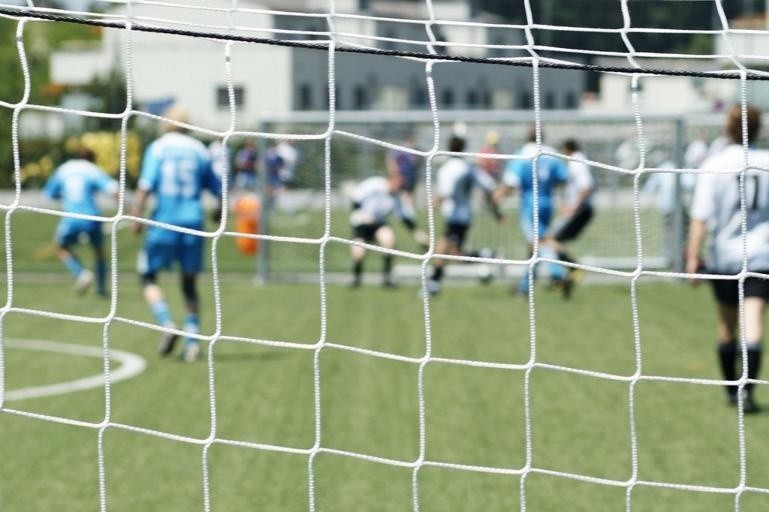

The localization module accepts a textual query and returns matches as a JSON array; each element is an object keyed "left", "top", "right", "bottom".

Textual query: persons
[
  {"left": 643, "top": 154, "right": 695, "bottom": 268},
  {"left": 206, "top": 133, "right": 298, "bottom": 193},
  {"left": 683, "top": 128, "right": 708, "bottom": 194},
  {"left": 344, "top": 133, "right": 600, "bottom": 293},
  {"left": 43, "top": 146, "right": 134, "bottom": 299},
  {"left": 134, "top": 107, "right": 229, "bottom": 364},
  {"left": 684, "top": 103, "right": 768, "bottom": 411}
]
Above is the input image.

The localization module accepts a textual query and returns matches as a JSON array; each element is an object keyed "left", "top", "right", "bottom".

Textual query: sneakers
[
  {"left": 350, "top": 250, "right": 578, "bottom": 299},
  {"left": 75, "top": 267, "right": 95, "bottom": 296},
  {"left": 158, "top": 324, "right": 201, "bottom": 362},
  {"left": 725, "top": 387, "right": 759, "bottom": 414}
]
